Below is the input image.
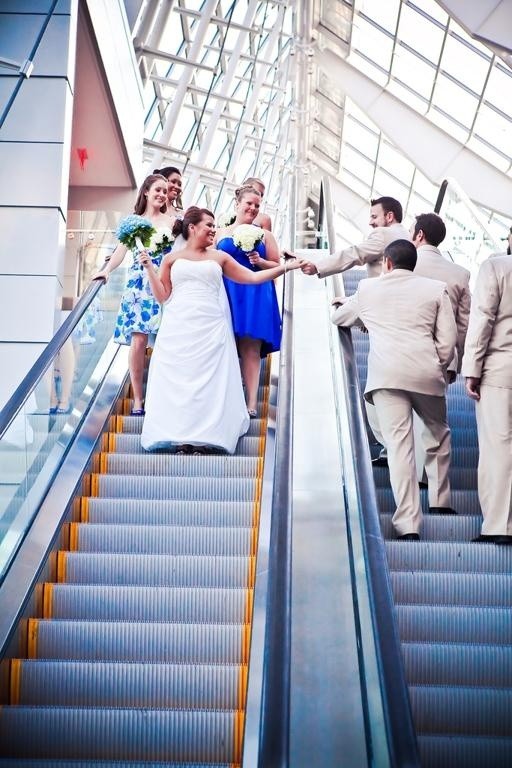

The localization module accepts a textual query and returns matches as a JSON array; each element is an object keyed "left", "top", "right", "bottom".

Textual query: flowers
[
  {"left": 115, "top": 214, "right": 156, "bottom": 265},
  {"left": 232, "top": 224, "right": 265, "bottom": 268},
  {"left": 217, "top": 210, "right": 237, "bottom": 228}
]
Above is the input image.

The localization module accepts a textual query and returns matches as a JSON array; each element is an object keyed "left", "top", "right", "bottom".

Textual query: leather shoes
[
  {"left": 372, "top": 458, "right": 458, "bottom": 539},
  {"left": 471, "top": 535, "right": 511, "bottom": 544}
]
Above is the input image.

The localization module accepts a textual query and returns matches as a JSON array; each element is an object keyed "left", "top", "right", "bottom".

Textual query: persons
[
  {"left": 461, "top": 228, "right": 511, "bottom": 544},
  {"left": 135, "top": 206, "right": 309, "bottom": 456},
  {"left": 222, "top": 177, "right": 272, "bottom": 231},
  {"left": 303, "top": 197, "right": 413, "bottom": 462},
  {"left": 50, "top": 337, "right": 79, "bottom": 415},
  {"left": 154, "top": 166, "right": 194, "bottom": 226},
  {"left": 330, "top": 239, "right": 457, "bottom": 541},
  {"left": 410, "top": 211, "right": 471, "bottom": 482},
  {"left": 93, "top": 174, "right": 187, "bottom": 414},
  {"left": 207, "top": 185, "right": 284, "bottom": 417},
  {"left": 52, "top": 288, "right": 104, "bottom": 382}
]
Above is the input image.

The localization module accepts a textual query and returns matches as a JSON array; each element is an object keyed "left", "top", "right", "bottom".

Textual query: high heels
[
  {"left": 47, "top": 369, "right": 77, "bottom": 414},
  {"left": 131, "top": 409, "right": 257, "bottom": 455}
]
